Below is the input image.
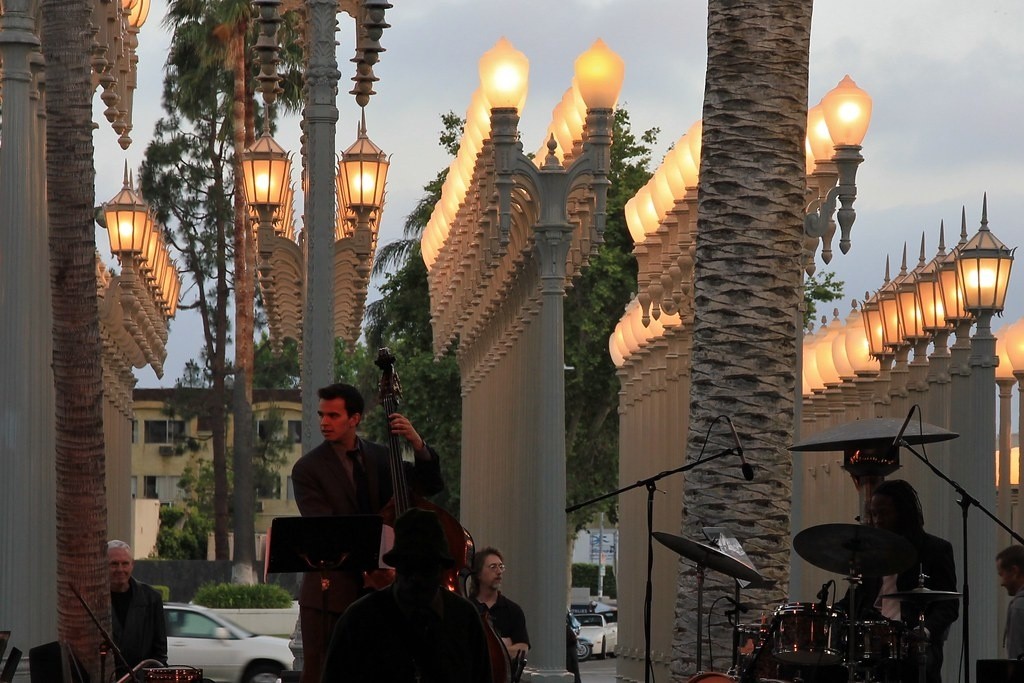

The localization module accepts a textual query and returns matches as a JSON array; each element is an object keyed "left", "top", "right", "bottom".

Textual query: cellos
[{"left": 321, "top": 348, "right": 511, "bottom": 683}]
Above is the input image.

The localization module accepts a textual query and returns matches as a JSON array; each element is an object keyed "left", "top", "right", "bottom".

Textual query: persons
[
  {"left": 292, "top": 384, "right": 445, "bottom": 683},
  {"left": 468, "top": 546, "right": 531, "bottom": 659},
  {"left": 995, "top": 544, "right": 1024, "bottom": 658},
  {"left": 321, "top": 509, "right": 493, "bottom": 683},
  {"left": 831, "top": 478, "right": 960, "bottom": 683},
  {"left": 107, "top": 540, "right": 169, "bottom": 683}
]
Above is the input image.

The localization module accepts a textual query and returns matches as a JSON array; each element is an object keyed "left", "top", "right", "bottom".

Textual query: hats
[{"left": 382, "top": 507, "right": 455, "bottom": 567}]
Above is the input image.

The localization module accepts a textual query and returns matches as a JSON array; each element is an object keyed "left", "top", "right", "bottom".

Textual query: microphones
[
  {"left": 816, "top": 580, "right": 832, "bottom": 599},
  {"left": 724, "top": 596, "right": 749, "bottom": 614},
  {"left": 726, "top": 416, "right": 753, "bottom": 481},
  {"left": 884, "top": 406, "right": 915, "bottom": 461}
]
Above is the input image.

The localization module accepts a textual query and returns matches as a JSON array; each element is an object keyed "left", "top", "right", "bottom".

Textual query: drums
[
  {"left": 686, "top": 672, "right": 739, "bottom": 683},
  {"left": 843, "top": 619, "right": 910, "bottom": 662},
  {"left": 738, "top": 626, "right": 800, "bottom": 683},
  {"left": 771, "top": 602, "right": 848, "bottom": 664}
]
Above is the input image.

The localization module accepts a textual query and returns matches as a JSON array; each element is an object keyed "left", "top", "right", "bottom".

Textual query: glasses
[{"left": 481, "top": 564, "right": 505, "bottom": 571}]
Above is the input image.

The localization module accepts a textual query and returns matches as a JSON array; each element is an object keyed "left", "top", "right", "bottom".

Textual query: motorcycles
[{"left": 572, "top": 616, "right": 591, "bottom": 661}]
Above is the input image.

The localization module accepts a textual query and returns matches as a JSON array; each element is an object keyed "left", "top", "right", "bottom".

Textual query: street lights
[
  {"left": 0, "top": 2, "right": 183, "bottom": 683},
  {"left": 243, "top": 109, "right": 391, "bottom": 452},
  {"left": 422, "top": 32, "right": 626, "bottom": 683},
  {"left": 608, "top": 74, "right": 875, "bottom": 683},
  {"left": 805, "top": 192, "right": 1023, "bottom": 683}
]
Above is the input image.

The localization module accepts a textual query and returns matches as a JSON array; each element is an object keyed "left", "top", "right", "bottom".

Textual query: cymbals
[
  {"left": 878, "top": 589, "right": 963, "bottom": 601},
  {"left": 793, "top": 523, "right": 917, "bottom": 575},
  {"left": 650, "top": 530, "right": 764, "bottom": 585}
]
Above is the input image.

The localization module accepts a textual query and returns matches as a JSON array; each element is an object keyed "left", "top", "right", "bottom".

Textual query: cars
[
  {"left": 575, "top": 613, "right": 618, "bottom": 659},
  {"left": 157, "top": 602, "right": 295, "bottom": 683}
]
